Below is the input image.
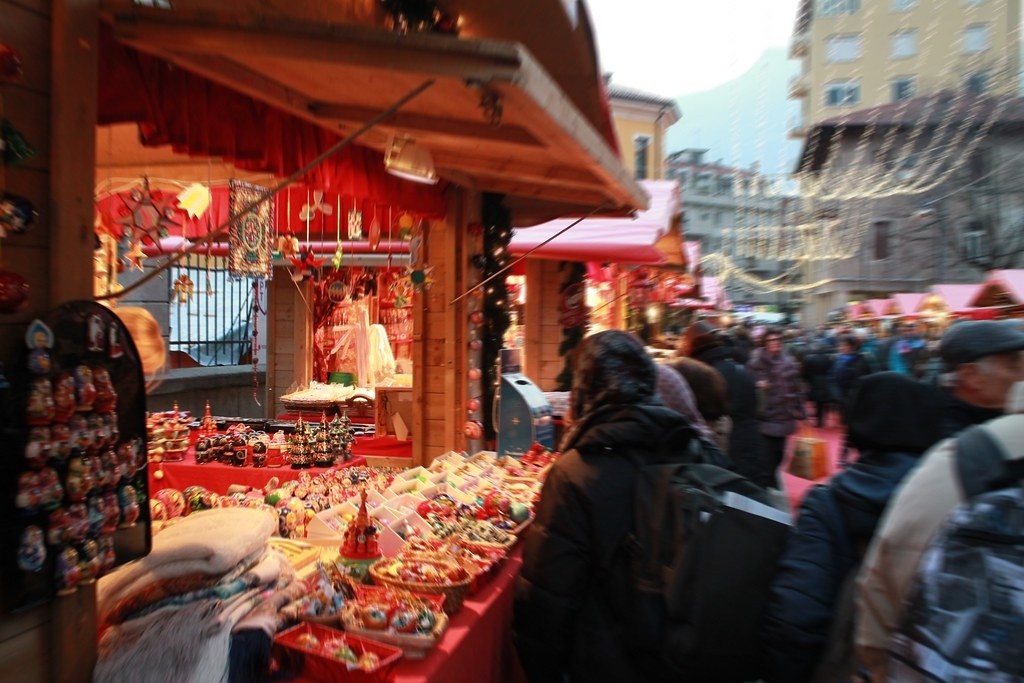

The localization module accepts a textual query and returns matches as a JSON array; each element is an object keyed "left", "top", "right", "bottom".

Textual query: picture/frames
[{"left": 226, "top": 178, "right": 274, "bottom": 282}]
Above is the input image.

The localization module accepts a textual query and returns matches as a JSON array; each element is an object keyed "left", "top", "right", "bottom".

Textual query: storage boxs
[
  {"left": 272, "top": 621, "right": 405, "bottom": 683},
  {"left": 308, "top": 448, "right": 498, "bottom": 553}
]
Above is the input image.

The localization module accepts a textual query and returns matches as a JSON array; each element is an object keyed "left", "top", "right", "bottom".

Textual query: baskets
[
  {"left": 475, "top": 489, "right": 536, "bottom": 533},
  {"left": 448, "top": 520, "right": 517, "bottom": 564},
  {"left": 273, "top": 620, "right": 402, "bottom": 683},
  {"left": 368, "top": 555, "right": 473, "bottom": 618},
  {"left": 342, "top": 595, "right": 448, "bottom": 660}
]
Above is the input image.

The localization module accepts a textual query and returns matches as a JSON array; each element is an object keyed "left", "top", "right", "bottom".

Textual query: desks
[
  {"left": 349, "top": 435, "right": 413, "bottom": 468},
  {"left": 147, "top": 447, "right": 365, "bottom": 499},
  {"left": 261, "top": 537, "right": 527, "bottom": 683}
]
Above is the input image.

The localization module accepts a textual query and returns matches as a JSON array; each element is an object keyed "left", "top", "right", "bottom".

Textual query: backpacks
[
  {"left": 885, "top": 424, "right": 1024, "bottom": 683},
  {"left": 572, "top": 427, "right": 797, "bottom": 683}
]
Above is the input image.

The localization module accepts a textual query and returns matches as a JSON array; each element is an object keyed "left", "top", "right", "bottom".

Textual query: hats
[
  {"left": 686, "top": 320, "right": 721, "bottom": 340},
  {"left": 936, "top": 319, "right": 1024, "bottom": 363}
]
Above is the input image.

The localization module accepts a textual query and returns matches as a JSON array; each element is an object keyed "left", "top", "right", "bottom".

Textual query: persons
[
  {"left": 515, "top": 317, "right": 1024, "bottom": 683},
  {"left": 516, "top": 330, "right": 706, "bottom": 683}
]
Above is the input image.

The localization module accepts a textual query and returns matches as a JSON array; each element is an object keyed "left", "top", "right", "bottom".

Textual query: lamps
[{"left": 382, "top": 132, "right": 441, "bottom": 187}]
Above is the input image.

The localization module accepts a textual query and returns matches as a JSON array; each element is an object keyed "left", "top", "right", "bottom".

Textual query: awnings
[{"left": 111, "top": 12, "right": 649, "bottom": 220}]
